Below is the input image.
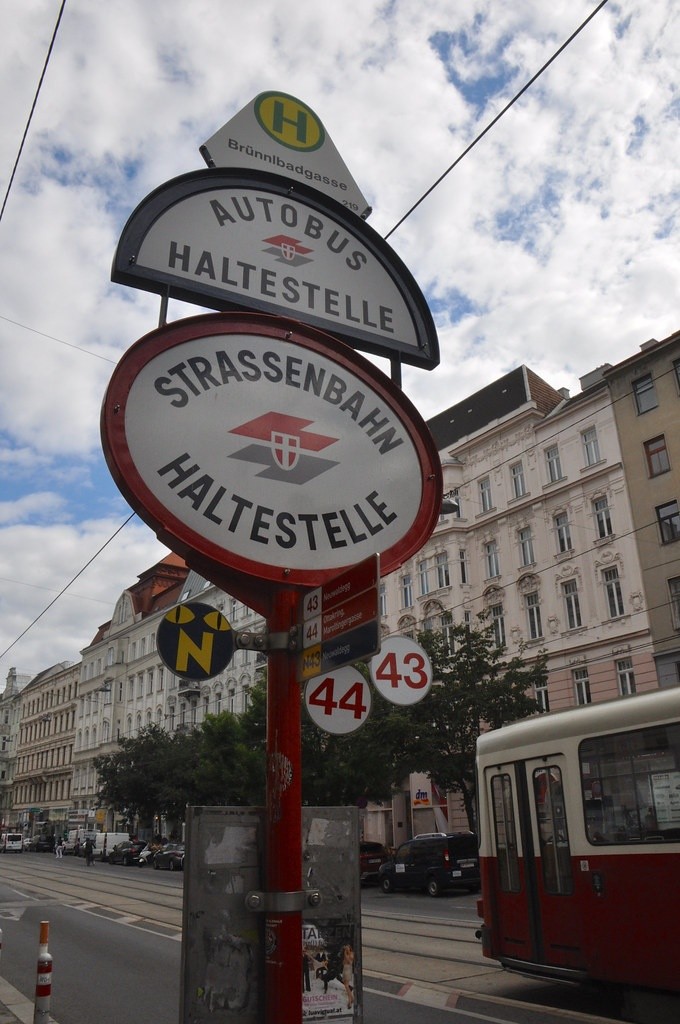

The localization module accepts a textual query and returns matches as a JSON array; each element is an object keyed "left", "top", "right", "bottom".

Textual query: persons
[
  {"left": 316, "top": 946, "right": 329, "bottom": 994},
  {"left": 56, "top": 837, "right": 63, "bottom": 859},
  {"left": 301, "top": 948, "right": 312, "bottom": 992},
  {"left": 83, "top": 838, "right": 96, "bottom": 866},
  {"left": 342, "top": 944, "right": 354, "bottom": 1009}
]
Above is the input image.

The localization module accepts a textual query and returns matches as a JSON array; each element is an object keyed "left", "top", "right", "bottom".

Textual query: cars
[
  {"left": 151, "top": 843, "right": 185, "bottom": 871},
  {"left": 107, "top": 839, "right": 148, "bottom": 867},
  {"left": 359, "top": 842, "right": 390, "bottom": 886},
  {"left": 29, "top": 835, "right": 53, "bottom": 852},
  {"left": 21, "top": 838, "right": 33, "bottom": 850}
]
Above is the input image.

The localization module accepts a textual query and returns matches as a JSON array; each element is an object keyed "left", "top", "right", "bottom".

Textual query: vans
[
  {"left": 5, "top": 833, "right": 22, "bottom": 853},
  {"left": 90, "top": 832, "right": 130, "bottom": 863}
]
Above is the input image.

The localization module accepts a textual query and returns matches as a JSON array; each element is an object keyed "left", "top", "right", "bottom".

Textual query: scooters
[{"left": 139, "top": 845, "right": 156, "bottom": 867}]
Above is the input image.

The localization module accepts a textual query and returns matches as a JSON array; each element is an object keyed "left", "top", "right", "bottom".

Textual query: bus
[{"left": 473, "top": 687, "right": 680, "bottom": 998}]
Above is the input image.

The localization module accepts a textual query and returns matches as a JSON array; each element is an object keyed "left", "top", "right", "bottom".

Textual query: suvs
[{"left": 379, "top": 830, "right": 483, "bottom": 896}]
